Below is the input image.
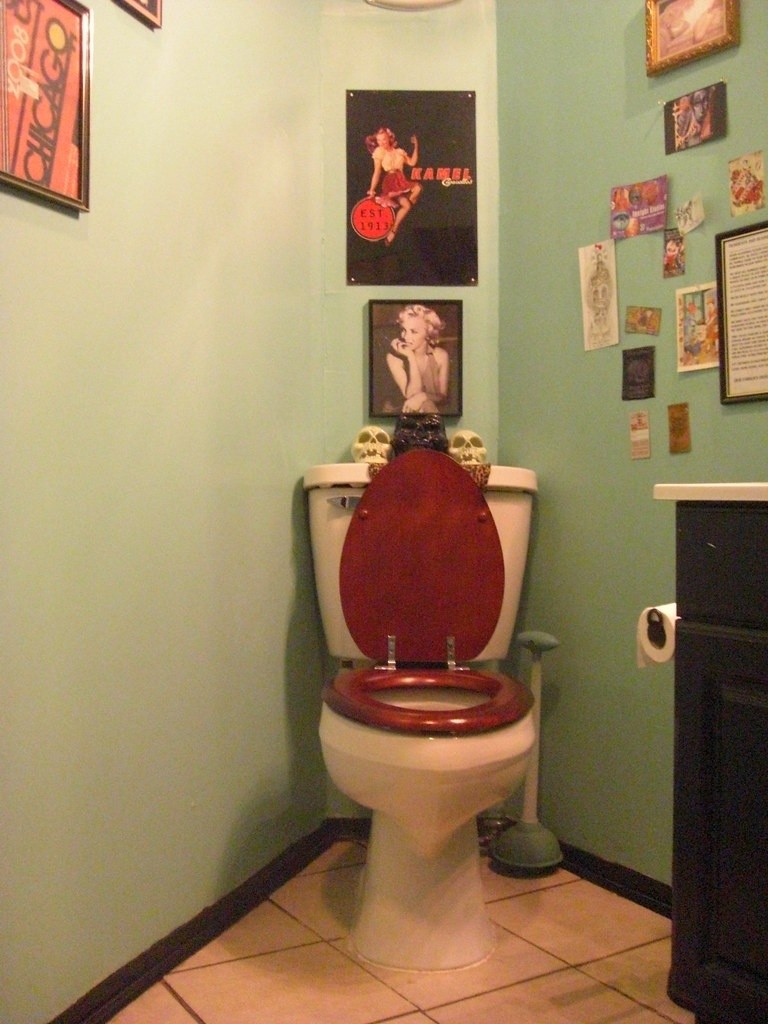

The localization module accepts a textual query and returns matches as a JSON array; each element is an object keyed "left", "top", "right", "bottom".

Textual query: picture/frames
[
  {"left": 714, "top": 217, "right": 768, "bottom": 405},
  {"left": 645, "top": 0, "right": 740, "bottom": 78},
  {"left": 367, "top": 299, "right": 464, "bottom": 418},
  {"left": 0, "top": 0, "right": 92, "bottom": 211},
  {"left": 115, "top": 0, "right": 164, "bottom": 28}
]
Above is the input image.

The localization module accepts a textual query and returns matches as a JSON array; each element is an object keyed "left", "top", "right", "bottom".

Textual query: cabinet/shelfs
[{"left": 667, "top": 501, "right": 768, "bottom": 1023}]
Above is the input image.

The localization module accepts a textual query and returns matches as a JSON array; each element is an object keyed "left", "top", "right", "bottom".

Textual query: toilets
[{"left": 304, "top": 464, "right": 536, "bottom": 971}]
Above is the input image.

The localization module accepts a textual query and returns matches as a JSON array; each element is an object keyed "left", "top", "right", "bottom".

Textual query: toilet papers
[{"left": 637, "top": 603, "right": 681, "bottom": 668}]
